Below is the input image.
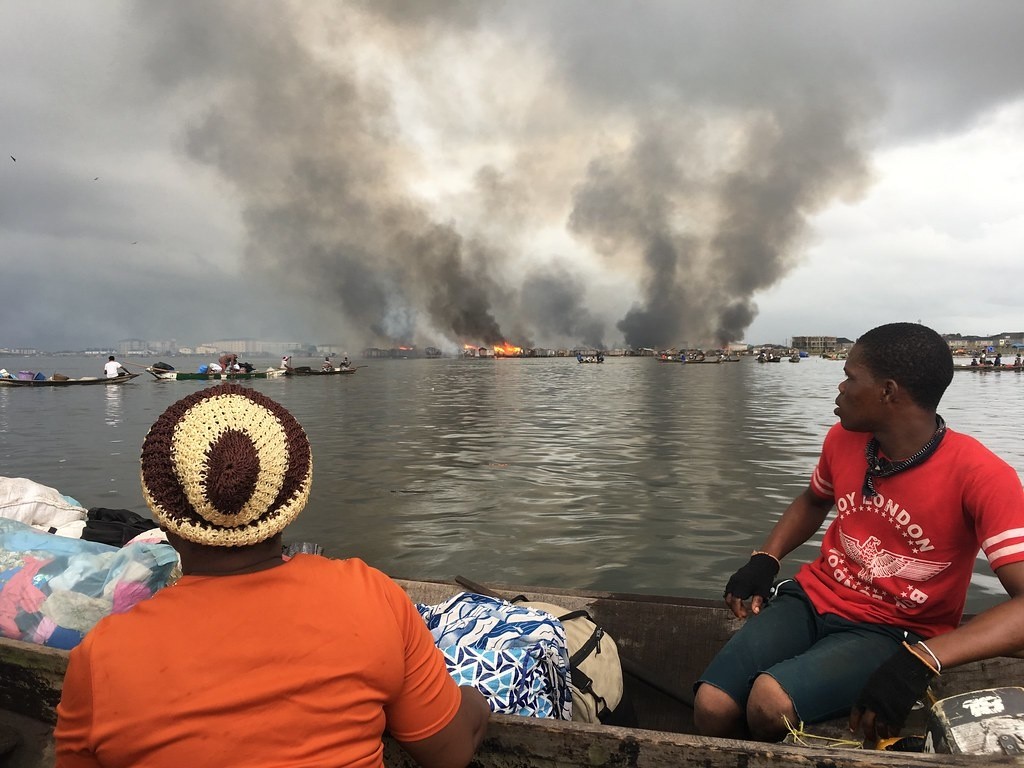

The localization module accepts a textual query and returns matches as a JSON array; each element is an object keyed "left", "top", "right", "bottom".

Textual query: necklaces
[{"left": 861, "top": 412, "right": 945, "bottom": 495}]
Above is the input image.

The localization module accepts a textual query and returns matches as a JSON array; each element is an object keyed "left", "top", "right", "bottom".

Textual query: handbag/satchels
[
  {"left": 414, "top": 591, "right": 572, "bottom": 724},
  {"left": 503, "top": 596, "right": 623, "bottom": 725},
  {"left": 80, "top": 508, "right": 159, "bottom": 548}
]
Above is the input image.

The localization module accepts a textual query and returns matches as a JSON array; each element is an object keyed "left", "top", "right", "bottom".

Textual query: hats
[{"left": 141, "top": 382, "right": 314, "bottom": 547}]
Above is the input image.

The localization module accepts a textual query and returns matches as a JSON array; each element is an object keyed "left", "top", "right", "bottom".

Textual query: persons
[
  {"left": 104, "top": 356, "right": 130, "bottom": 379},
  {"left": 576, "top": 322, "right": 1024, "bottom": 741},
  {"left": 340, "top": 357, "right": 351, "bottom": 371},
  {"left": 207, "top": 350, "right": 252, "bottom": 374},
  {"left": 281, "top": 356, "right": 292, "bottom": 370},
  {"left": 53, "top": 382, "right": 491, "bottom": 768},
  {"left": 321, "top": 357, "right": 335, "bottom": 372}
]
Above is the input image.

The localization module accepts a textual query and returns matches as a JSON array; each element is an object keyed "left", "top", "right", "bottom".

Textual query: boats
[
  {"left": 578, "top": 360, "right": 603, "bottom": 363},
  {"left": 754, "top": 352, "right": 848, "bottom": 363},
  {"left": 0, "top": 563, "right": 1024, "bottom": 768},
  {"left": 1, "top": 371, "right": 144, "bottom": 386},
  {"left": 952, "top": 363, "right": 1024, "bottom": 372},
  {"left": 278, "top": 365, "right": 358, "bottom": 376},
  {"left": 656, "top": 358, "right": 740, "bottom": 363},
  {"left": 145, "top": 361, "right": 286, "bottom": 380}
]
